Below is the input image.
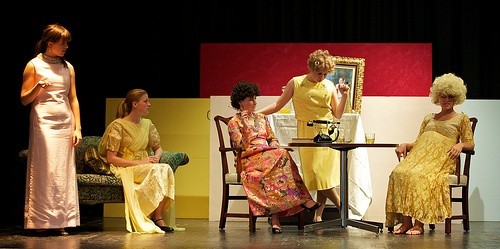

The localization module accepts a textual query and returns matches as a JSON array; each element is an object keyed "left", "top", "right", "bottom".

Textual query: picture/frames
[{"left": 325, "top": 56, "right": 366, "bottom": 113}]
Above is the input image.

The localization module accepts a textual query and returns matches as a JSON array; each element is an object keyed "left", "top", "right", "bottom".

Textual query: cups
[{"left": 365, "top": 133, "right": 375, "bottom": 144}]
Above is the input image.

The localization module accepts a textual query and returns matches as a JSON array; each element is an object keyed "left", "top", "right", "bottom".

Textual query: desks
[
  {"left": 268, "top": 113, "right": 374, "bottom": 221},
  {"left": 287, "top": 139, "right": 399, "bottom": 233}
]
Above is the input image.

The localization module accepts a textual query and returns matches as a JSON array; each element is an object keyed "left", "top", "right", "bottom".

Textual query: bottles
[{"left": 277, "top": 86, "right": 292, "bottom": 114}]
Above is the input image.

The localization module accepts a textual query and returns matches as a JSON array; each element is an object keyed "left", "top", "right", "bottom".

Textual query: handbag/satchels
[{"left": 85, "top": 147, "right": 112, "bottom": 176}]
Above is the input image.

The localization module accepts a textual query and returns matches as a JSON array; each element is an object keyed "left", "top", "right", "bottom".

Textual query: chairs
[
  {"left": 428, "top": 116, "right": 478, "bottom": 235},
  {"left": 213, "top": 116, "right": 258, "bottom": 233}
]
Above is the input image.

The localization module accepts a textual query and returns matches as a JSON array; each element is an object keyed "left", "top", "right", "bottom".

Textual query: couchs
[{"left": 19, "top": 136, "right": 189, "bottom": 203}]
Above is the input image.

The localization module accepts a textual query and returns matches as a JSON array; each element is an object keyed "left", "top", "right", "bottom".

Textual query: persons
[
  {"left": 99, "top": 89, "right": 175, "bottom": 234},
  {"left": 257, "top": 50, "right": 350, "bottom": 222},
  {"left": 386, "top": 73, "right": 475, "bottom": 234},
  {"left": 20, "top": 24, "right": 82, "bottom": 236},
  {"left": 228, "top": 81, "right": 322, "bottom": 234},
  {"left": 336, "top": 77, "right": 348, "bottom": 100}
]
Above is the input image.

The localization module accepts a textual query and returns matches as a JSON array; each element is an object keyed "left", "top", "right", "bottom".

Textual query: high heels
[
  {"left": 300, "top": 200, "right": 322, "bottom": 212},
  {"left": 270, "top": 223, "right": 283, "bottom": 233}
]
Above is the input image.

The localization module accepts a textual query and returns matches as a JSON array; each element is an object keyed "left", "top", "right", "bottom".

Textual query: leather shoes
[{"left": 154, "top": 219, "right": 174, "bottom": 231}]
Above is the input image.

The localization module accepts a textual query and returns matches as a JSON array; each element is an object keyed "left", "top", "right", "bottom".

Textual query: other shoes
[{"left": 59, "top": 229, "right": 68, "bottom": 236}]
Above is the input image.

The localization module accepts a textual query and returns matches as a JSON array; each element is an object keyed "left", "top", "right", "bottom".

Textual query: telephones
[{"left": 306, "top": 118, "right": 340, "bottom": 144}]
